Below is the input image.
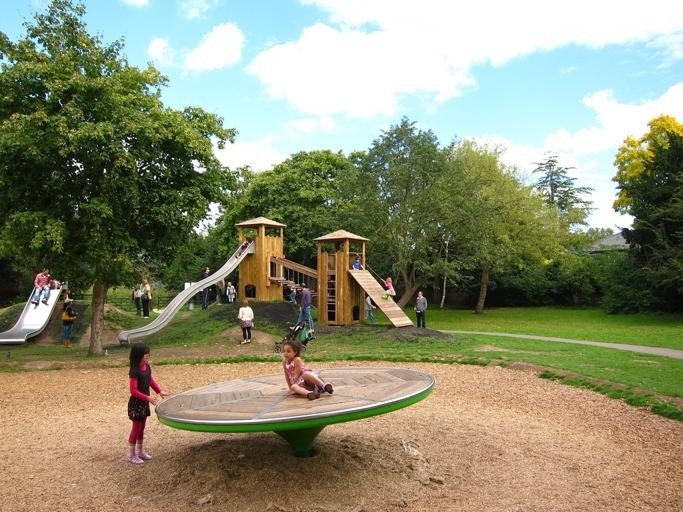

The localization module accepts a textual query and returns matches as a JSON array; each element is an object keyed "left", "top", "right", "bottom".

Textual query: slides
[
  {"left": 118, "top": 240, "right": 256, "bottom": 346},
  {"left": 0, "top": 281, "right": 63, "bottom": 345}
]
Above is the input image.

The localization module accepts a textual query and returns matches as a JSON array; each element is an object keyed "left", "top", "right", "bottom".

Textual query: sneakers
[
  {"left": 31, "top": 300, "right": 38, "bottom": 304},
  {"left": 128, "top": 456, "right": 144, "bottom": 464},
  {"left": 137, "top": 453, "right": 152, "bottom": 460},
  {"left": 307, "top": 392, "right": 320, "bottom": 399},
  {"left": 324, "top": 384, "right": 333, "bottom": 394},
  {"left": 42, "top": 299, "right": 47, "bottom": 303}
]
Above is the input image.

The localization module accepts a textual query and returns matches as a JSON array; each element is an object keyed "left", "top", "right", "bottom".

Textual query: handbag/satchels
[{"left": 62, "top": 312, "right": 76, "bottom": 320}]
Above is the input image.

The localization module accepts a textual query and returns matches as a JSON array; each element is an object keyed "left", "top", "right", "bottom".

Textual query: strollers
[{"left": 272, "top": 319, "right": 314, "bottom": 354}]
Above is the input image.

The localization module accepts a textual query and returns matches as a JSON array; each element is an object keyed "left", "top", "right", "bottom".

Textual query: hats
[{"left": 65, "top": 299, "right": 73, "bottom": 303}]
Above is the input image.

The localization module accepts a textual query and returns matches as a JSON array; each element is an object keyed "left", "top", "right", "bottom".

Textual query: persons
[
  {"left": 411, "top": 292, "right": 428, "bottom": 329},
  {"left": 364, "top": 295, "right": 376, "bottom": 321},
  {"left": 62, "top": 299, "right": 79, "bottom": 348},
  {"left": 351, "top": 255, "right": 364, "bottom": 270},
  {"left": 200, "top": 266, "right": 212, "bottom": 310},
  {"left": 234, "top": 240, "right": 248, "bottom": 260},
  {"left": 140, "top": 278, "right": 151, "bottom": 319},
  {"left": 48, "top": 278, "right": 61, "bottom": 290},
  {"left": 226, "top": 282, "right": 236, "bottom": 303},
  {"left": 288, "top": 282, "right": 315, "bottom": 334},
  {"left": 380, "top": 277, "right": 396, "bottom": 300},
  {"left": 30, "top": 267, "right": 51, "bottom": 305},
  {"left": 281, "top": 339, "right": 333, "bottom": 401},
  {"left": 124, "top": 342, "right": 171, "bottom": 465},
  {"left": 236, "top": 298, "right": 255, "bottom": 345},
  {"left": 132, "top": 285, "right": 142, "bottom": 317},
  {"left": 288, "top": 281, "right": 297, "bottom": 304}
]
